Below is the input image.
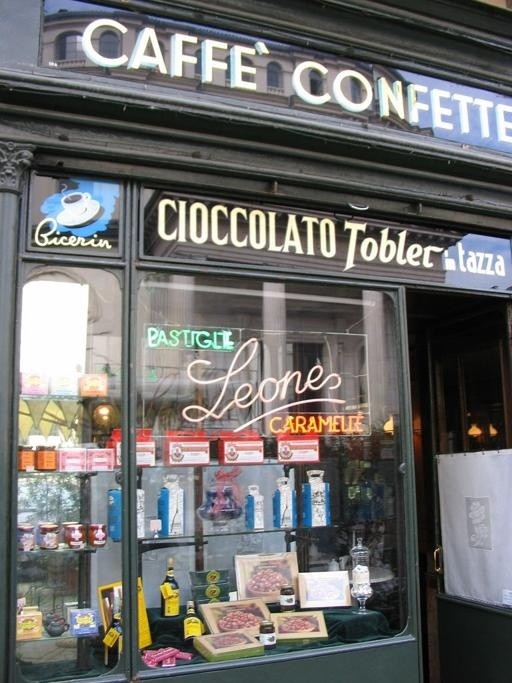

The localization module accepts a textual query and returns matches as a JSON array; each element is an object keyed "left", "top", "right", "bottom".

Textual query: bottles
[
  {"left": 184, "top": 600, "right": 200, "bottom": 639},
  {"left": 38, "top": 525, "right": 59, "bottom": 550},
  {"left": 17, "top": 526, "right": 33, "bottom": 551},
  {"left": 260, "top": 622, "right": 276, "bottom": 649},
  {"left": 66, "top": 525, "right": 86, "bottom": 549},
  {"left": 36, "top": 444, "right": 57, "bottom": 470},
  {"left": 18, "top": 445, "right": 36, "bottom": 470},
  {"left": 107, "top": 602, "right": 122, "bottom": 668},
  {"left": 160, "top": 557, "right": 178, "bottom": 619},
  {"left": 88, "top": 525, "right": 108, "bottom": 546},
  {"left": 280, "top": 586, "right": 295, "bottom": 612}
]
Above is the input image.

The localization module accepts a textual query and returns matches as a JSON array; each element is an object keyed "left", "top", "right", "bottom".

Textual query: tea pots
[{"left": 43, "top": 609, "right": 69, "bottom": 636}]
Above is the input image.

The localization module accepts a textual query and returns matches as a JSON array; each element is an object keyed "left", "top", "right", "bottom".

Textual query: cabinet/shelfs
[
  {"left": 137, "top": 265, "right": 408, "bottom": 672},
  {"left": 17, "top": 262, "right": 125, "bottom": 680}
]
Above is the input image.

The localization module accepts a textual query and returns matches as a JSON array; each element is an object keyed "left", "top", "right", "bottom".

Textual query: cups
[
  {"left": 39, "top": 526, "right": 60, "bottom": 550},
  {"left": 89, "top": 524, "right": 108, "bottom": 547},
  {"left": 17, "top": 522, "right": 31, "bottom": 526},
  {"left": 66, "top": 525, "right": 87, "bottom": 550},
  {"left": 18, "top": 526, "right": 34, "bottom": 551},
  {"left": 39, "top": 521, "right": 53, "bottom": 526},
  {"left": 63, "top": 521, "right": 79, "bottom": 541}
]
